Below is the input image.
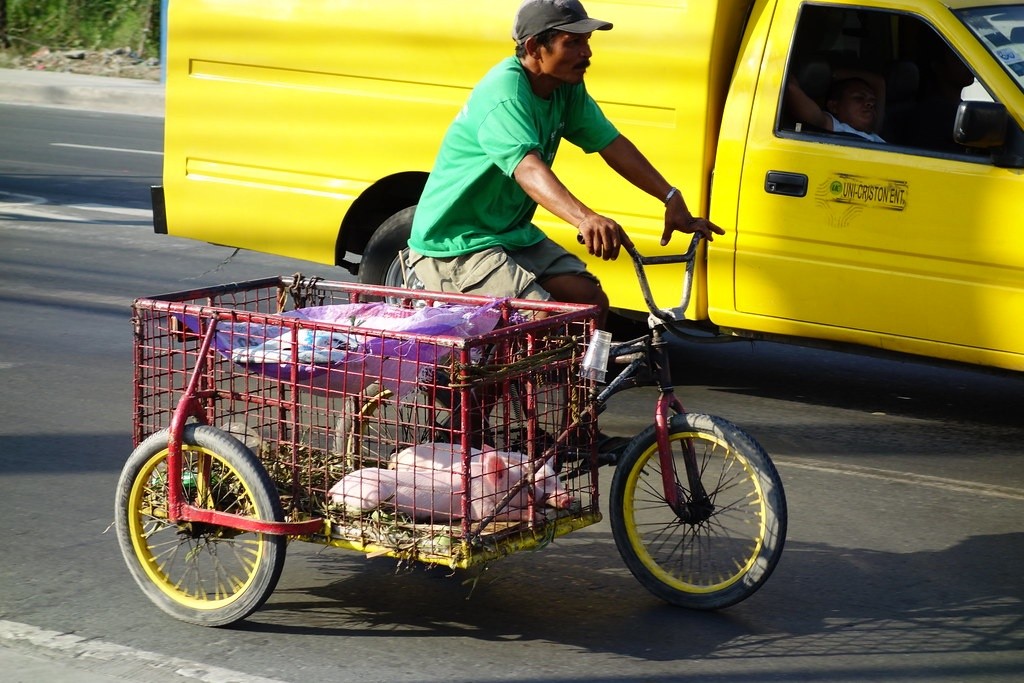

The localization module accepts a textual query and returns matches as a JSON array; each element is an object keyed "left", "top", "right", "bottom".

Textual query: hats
[{"left": 514, "top": 0, "right": 614, "bottom": 45}]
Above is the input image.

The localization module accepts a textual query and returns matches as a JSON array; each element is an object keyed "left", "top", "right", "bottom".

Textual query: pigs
[
  {"left": 326, "top": 443, "right": 551, "bottom": 523},
  {"left": 386, "top": 443, "right": 574, "bottom": 509}
]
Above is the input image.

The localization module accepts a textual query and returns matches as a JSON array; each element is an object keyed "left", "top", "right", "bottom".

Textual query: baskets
[{"left": 133, "top": 274, "right": 601, "bottom": 566}]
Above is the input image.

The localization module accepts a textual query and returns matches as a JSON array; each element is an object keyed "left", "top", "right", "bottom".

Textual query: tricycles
[{"left": 113, "top": 224, "right": 786, "bottom": 628}]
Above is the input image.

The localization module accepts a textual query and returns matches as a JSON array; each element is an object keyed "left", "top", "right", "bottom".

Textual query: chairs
[
  {"left": 784, "top": 58, "right": 835, "bottom": 134},
  {"left": 883, "top": 61, "right": 920, "bottom": 143}
]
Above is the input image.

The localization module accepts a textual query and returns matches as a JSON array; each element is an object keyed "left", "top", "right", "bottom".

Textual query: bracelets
[{"left": 663, "top": 187, "right": 678, "bottom": 207}]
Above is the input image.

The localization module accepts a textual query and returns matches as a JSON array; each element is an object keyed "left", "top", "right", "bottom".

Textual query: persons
[
  {"left": 778, "top": 66, "right": 890, "bottom": 144},
  {"left": 393, "top": 3, "right": 728, "bottom": 471},
  {"left": 926, "top": 44, "right": 976, "bottom": 122}
]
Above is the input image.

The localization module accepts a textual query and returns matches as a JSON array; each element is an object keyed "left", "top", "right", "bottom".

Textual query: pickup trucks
[{"left": 153, "top": 0, "right": 1024, "bottom": 383}]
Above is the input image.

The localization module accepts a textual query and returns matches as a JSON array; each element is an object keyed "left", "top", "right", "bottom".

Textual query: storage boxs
[{"left": 131, "top": 276, "right": 603, "bottom": 569}]
